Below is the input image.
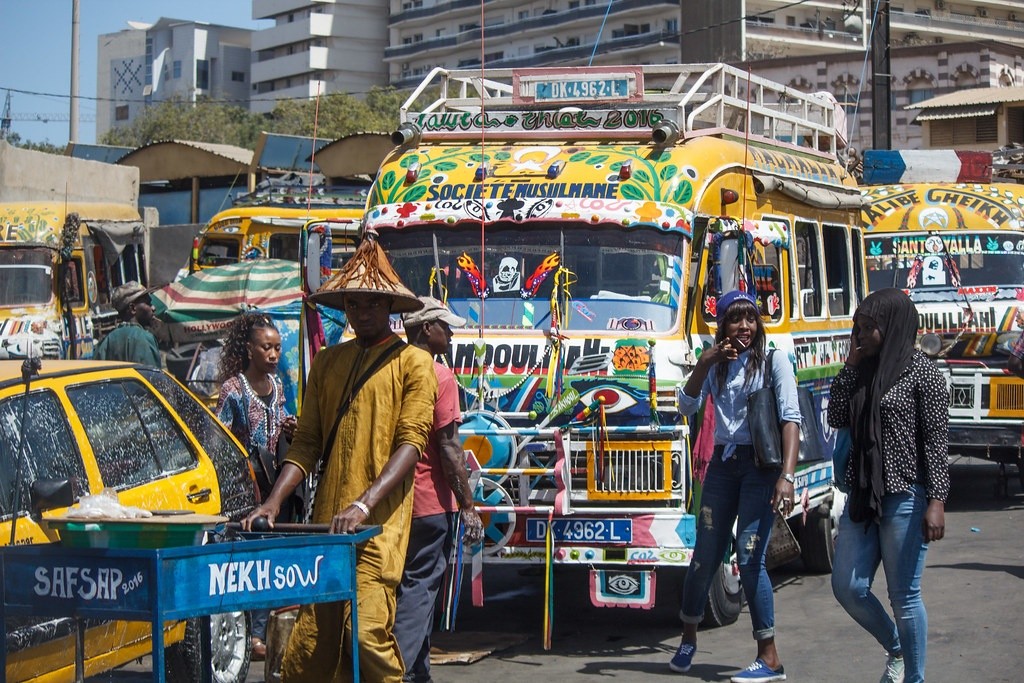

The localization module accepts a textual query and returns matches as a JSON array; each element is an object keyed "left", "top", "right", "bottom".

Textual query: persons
[
  {"left": 94, "top": 280, "right": 162, "bottom": 371},
  {"left": 393, "top": 296, "right": 484, "bottom": 683},
  {"left": 241, "top": 294, "right": 439, "bottom": 683},
  {"left": 215, "top": 310, "right": 298, "bottom": 661},
  {"left": 826, "top": 290, "right": 951, "bottom": 683},
  {"left": 669, "top": 292, "right": 804, "bottom": 683}
]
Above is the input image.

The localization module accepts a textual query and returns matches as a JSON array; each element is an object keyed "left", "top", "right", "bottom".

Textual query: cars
[{"left": 0, "top": 356, "right": 261, "bottom": 683}]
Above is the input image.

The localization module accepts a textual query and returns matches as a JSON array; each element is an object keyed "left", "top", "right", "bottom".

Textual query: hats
[
  {"left": 111, "top": 280, "right": 158, "bottom": 313},
  {"left": 309, "top": 233, "right": 425, "bottom": 314},
  {"left": 716, "top": 290, "right": 760, "bottom": 323},
  {"left": 403, "top": 296, "right": 467, "bottom": 327}
]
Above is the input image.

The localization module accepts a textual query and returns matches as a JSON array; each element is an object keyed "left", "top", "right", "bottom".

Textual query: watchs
[{"left": 780, "top": 473, "right": 794, "bottom": 483}]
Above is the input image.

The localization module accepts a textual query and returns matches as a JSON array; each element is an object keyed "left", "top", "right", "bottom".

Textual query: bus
[{"left": 0, "top": 62, "right": 1024, "bottom": 631}]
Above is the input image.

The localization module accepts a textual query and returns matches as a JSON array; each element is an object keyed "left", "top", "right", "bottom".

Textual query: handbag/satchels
[
  {"left": 833, "top": 427, "right": 852, "bottom": 493},
  {"left": 747, "top": 348, "right": 825, "bottom": 469}
]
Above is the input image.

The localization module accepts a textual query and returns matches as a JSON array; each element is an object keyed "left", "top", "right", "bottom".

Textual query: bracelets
[
  {"left": 844, "top": 362, "right": 856, "bottom": 368},
  {"left": 352, "top": 501, "right": 371, "bottom": 518}
]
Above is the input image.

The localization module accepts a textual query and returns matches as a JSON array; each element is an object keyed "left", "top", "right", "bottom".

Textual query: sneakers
[
  {"left": 880, "top": 653, "right": 905, "bottom": 683},
  {"left": 731, "top": 658, "right": 786, "bottom": 683},
  {"left": 669, "top": 641, "right": 697, "bottom": 673}
]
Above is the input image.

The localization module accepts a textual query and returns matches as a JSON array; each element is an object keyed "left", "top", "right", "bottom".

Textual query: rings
[
  {"left": 784, "top": 497, "right": 790, "bottom": 501},
  {"left": 720, "top": 341, "right": 723, "bottom": 346}
]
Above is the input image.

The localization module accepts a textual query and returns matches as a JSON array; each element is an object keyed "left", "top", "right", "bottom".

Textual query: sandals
[{"left": 251, "top": 638, "right": 266, "bottom": 660}]
